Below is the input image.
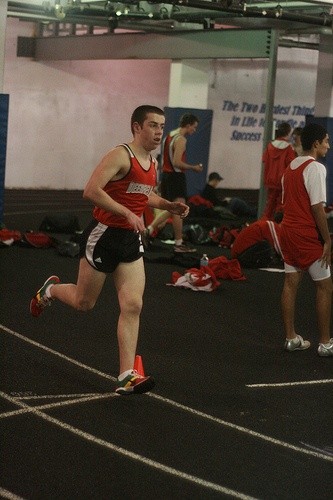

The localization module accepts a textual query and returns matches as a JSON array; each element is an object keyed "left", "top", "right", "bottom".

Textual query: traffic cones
[{"left": 134, "top": 355, "right": 145, "bottom": 378}]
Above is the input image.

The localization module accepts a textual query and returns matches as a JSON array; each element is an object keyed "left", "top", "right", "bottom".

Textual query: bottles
[{"left": 200, "top": 254, "right": 208, "bottom": 266}]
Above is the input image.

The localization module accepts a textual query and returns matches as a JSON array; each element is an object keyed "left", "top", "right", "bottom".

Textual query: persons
[
  {"left": 290, "top": 127, "right": 304, "bottom": 156},
  {"left": 232, "top": 210, "right": 285, "bottom": 269},
  {"left": 30, "top": 105, "right": 189, "bottom": 396},
  {"left": 281, "top": 123, "right": 333, "bottom": 357},
  {"left": 263, "top": 123, "right": 298, "bottom": 221},
  {"left": 141, "top": 113, "right": 198, "bottom": 252},
  {"left": 201, "top": 173, "right": 257, "bottom": 220}
]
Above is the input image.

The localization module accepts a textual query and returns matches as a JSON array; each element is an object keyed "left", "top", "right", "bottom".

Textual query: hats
[{"left": 208, "top": 172, "right": 223, "bottom": 181}]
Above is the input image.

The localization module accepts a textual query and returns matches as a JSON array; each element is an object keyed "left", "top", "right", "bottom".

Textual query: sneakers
[
  {"left": 174, "top": 242, "right": 196, "bottom": 252},
  {"left": 111, "top": 370, "right": 155, "bottom": 401},
  {"left": 29, "top": 275, "right": 60, "bottom": 318},
  {"left": 317, "top": 338, "right": 332, "bottom": 356},
  {"left": 284, "top": 334, "right": 311, "bottom": 351},
  {"left": 141, "top": 229, "right": 149, "bottom": 248}
]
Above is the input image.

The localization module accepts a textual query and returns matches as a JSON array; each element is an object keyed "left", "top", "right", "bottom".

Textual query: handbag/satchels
[{"left": 34, "top": 211, "right": 80, "bottom": 234}]
